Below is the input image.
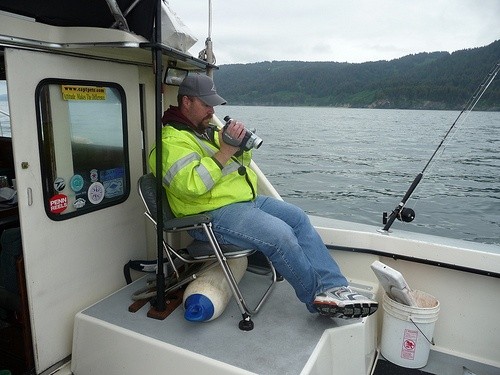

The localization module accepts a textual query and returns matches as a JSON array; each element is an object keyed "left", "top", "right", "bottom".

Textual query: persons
[{"left": 148, "top": 72, "right": 378, "bottom": 320}]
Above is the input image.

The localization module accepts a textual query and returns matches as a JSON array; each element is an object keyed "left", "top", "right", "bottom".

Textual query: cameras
[{"left": 223, "top": 115, "right": 263, "bottom": 150}]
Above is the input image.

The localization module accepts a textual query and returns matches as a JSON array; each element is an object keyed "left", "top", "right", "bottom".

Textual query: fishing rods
[{"left": 383, "top": 62, "right": 500, "bottom": 232}]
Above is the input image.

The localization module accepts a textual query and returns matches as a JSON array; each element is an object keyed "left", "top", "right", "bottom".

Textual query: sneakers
[{"left": 312, "top": 286, "right": 379, "bottom": 319}]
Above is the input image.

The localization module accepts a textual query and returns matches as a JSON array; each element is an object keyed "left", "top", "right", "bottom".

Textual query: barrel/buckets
[{"left": 380, "top": 287, "right": 440, "bottom": 369}]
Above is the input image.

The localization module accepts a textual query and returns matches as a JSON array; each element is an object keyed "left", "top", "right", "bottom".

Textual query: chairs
[{"left": 138, "top": 173, "right": 283, "bottom": 331}]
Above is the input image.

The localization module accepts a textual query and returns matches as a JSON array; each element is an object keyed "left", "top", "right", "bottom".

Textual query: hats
[{"left": 177, "top": 73, "right": 227, "bottom": 106}]
